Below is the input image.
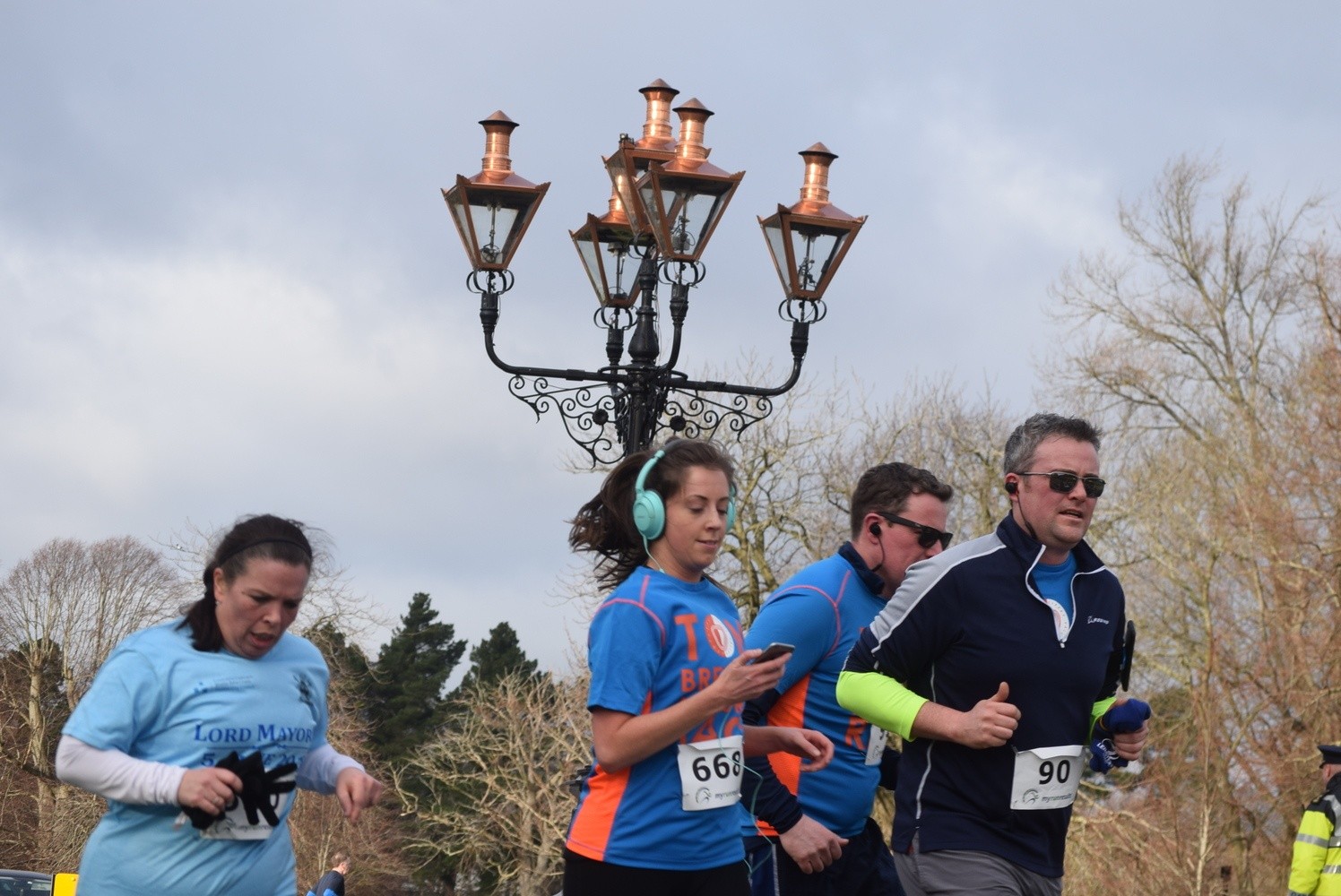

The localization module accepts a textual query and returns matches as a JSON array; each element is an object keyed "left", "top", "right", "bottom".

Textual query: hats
[{"left": 1316, "top": 745, "right": 1341, "bottom": 769}]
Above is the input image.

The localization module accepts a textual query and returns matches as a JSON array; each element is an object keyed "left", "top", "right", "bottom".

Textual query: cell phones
[
  {"left": 1121, "top": 620, "right": 1135, "bottom": 692},
  {"left": 751, "top": 643, "right": 795, "bottom": 664}
]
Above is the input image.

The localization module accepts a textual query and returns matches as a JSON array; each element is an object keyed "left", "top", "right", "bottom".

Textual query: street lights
[{"left": 439, "top": 79, "right": 870, "bottom": 575}]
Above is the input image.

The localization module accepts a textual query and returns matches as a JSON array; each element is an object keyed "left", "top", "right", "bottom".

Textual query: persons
[
  {"left": 744, "top": 462, "right": 954, "bottom": 895},
  {"left": 1287, "top": 744, "right": 1341, "bottom": 896},
  {"left": 54, "top": 513, "right": 387, "bottom": 896},
  {"left": 835, "top": 413, "right": 1152, "bottom": 896},
  {"left": 305, "top": 851, "right": 353, "bottom": 896},
  {"left": 562, "top": 435, "right": 836, "bottom": 896}
]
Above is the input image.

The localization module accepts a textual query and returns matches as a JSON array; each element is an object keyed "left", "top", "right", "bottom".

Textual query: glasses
[
  {"left": 1018, "top": 472, "right": 1106, "bottom": 498},
  {"left": 877, "top": 511, "right": 953, "bottom": 551}
]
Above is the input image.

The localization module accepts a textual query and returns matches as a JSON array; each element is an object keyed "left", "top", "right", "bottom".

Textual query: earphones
[
  {"left": 869, "top": 524, "right": 881, "bottom": 535},
  {"left": 1005, "top": 482, "right": 1018, "bottom": 494}
]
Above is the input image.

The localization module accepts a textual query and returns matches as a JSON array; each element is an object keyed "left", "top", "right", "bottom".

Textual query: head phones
[{"left": 633, "top": 439, "right": 735, "bottom": 542}]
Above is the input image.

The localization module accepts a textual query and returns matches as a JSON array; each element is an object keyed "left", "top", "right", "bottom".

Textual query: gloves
[
  {"left": 182, "top": 751, "right": 298, "bottom": 830},
  {"left": 1089, "top": 699, "right": 1151, "bottom": 774}
]
